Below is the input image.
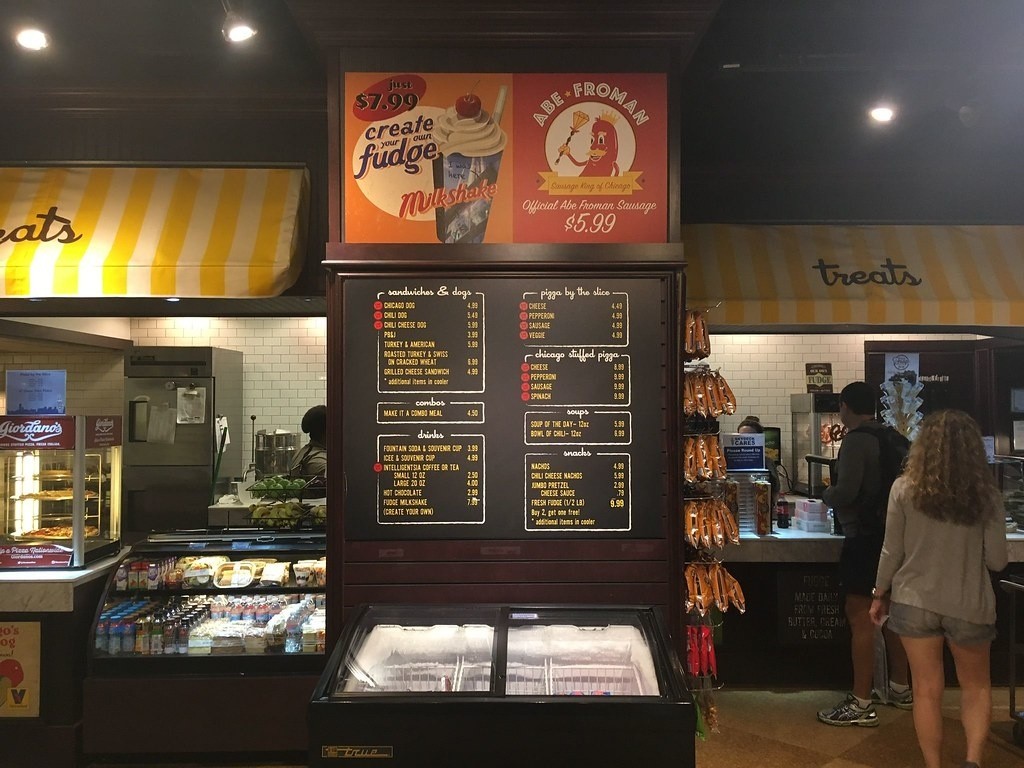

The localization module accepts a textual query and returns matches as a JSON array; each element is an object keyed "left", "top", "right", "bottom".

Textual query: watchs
[{"left": 870, "top": 586, "right": 888, "bottom": 603}]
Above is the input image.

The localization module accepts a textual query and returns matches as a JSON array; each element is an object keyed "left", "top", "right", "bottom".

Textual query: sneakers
[
  {"left": 872, "top": 687, "right": 914, "bottom": 711},
  {"left": 815, "top": 694, "right": 880, "bottom": 727}
]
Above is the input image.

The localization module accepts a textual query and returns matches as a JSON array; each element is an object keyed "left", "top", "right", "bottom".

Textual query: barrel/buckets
[{"left": 252, "top": 428, "right": 302, "bottom": 481}]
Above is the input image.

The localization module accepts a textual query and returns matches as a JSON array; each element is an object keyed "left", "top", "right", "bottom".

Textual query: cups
[{"left": 427, "top": 135, "right": 501, "bottom": 244}]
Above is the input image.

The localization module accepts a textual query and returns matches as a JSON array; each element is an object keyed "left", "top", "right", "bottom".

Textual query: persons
[
  {"left": 736, "top": 412, "right": 782, "bottom": 515},
  {"left": 285, "top": 404, "right": 328, "bottom": 487},
  {"left": 865, "top": 407, "right": 1013, "bottom": 768},
  {"left": 813, "top": 380, "right": 918, "bottom": 727}
]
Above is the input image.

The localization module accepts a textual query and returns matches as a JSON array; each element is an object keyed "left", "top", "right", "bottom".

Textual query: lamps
[
  {"left": 864, "top": 66, "right": 904, "bottom": 126},
  {"left": 10, "top": 0, "right": 56, "bottom": 56},
  {"left": 220, "top": 0, "right": 260, "bottom": 44}
]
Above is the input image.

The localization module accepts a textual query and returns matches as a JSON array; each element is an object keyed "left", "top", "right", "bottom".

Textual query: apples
[{"left": 251, "top": 475, "right": 306, "bottom": 498}]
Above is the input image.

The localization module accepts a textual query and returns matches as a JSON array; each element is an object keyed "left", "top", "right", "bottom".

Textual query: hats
[{"left": 301, "top": 406, "right": 327, "bottom": 432}]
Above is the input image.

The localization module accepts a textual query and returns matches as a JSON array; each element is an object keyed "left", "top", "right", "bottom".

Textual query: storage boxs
[{"left": 242, "top": 474, "right": 327, "bottom": 532}]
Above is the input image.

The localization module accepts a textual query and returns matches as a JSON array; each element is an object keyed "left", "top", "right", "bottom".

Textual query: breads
[{"left": 188, "top": 607, "right": 325, "bottom": 654}]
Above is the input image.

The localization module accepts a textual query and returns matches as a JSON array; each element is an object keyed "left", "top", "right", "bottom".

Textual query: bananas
[{"left": 249, "top": 502, "right": 325, "bottom": 527}]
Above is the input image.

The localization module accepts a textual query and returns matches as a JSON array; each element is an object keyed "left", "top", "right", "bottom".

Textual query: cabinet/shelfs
[
  {"left": 0, "top": 414, "right": 125, "bottom": 568},
  {"left": 79, "top": 539, "right": 330, "bottom": 754}
]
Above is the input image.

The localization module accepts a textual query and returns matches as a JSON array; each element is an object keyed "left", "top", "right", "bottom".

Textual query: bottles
[
  {"left": 776, "top": 491, "right": 789, "bottom": 528},
  {"left": 93, "top": 555, "right": 328, "bottom": 657}
]
